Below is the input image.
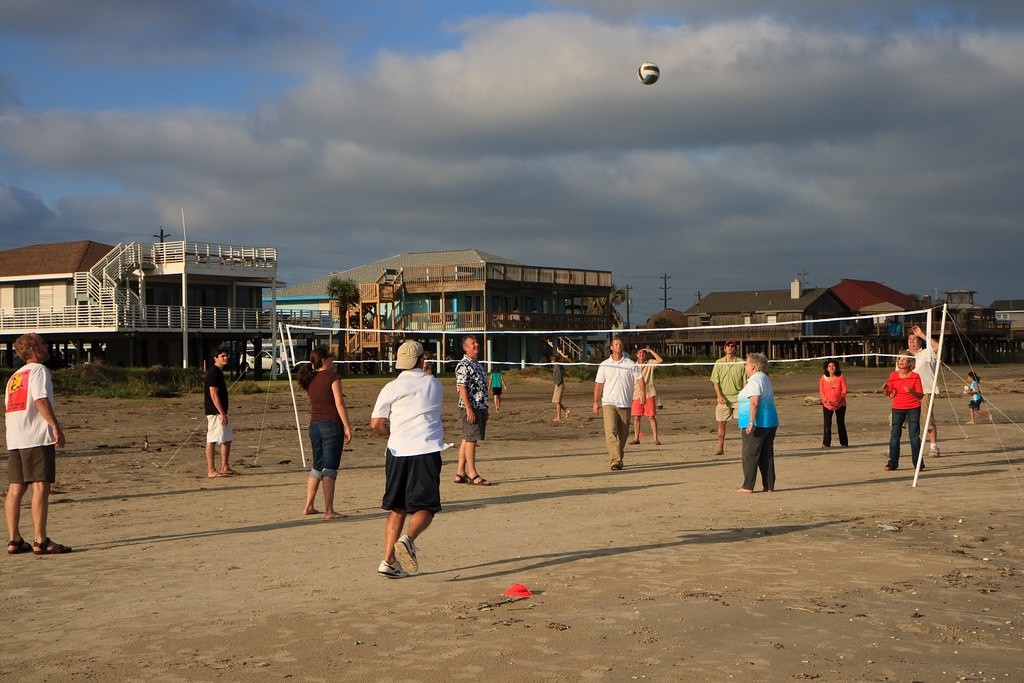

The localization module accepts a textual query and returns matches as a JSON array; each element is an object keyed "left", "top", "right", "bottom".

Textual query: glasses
[
  {"left": 327, "top": 354, "right": 332, "bottom": 358},
  {"left": 726, "top": 345, "right": 736, "bottom": 347}
]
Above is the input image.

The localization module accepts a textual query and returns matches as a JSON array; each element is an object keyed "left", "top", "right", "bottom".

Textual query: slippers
[
  {"left": 220, "top": 470, "right": 241, "bottom": 475},
  {"left": 208, "top": 473, "right": 232, "bottom": 478}
]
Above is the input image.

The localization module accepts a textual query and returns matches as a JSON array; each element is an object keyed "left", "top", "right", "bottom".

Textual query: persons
[
  {"left": 709, "top": 338, "right": 779, "bottom": 493},
  {"left": 592, "top": 337, "right": 646, "bottom": 470},
  {"left": 296, "top": 347, "right": 352, "bottom": 520},
  {"left": 204, "top": 348, "right": 241, "bottom": 478},
  {"left": 629, "top": 346, "right": 663, "bottom": 445},
  {"left": 454, "top": 335, "right": 491, "bottom": 486},
  {"left": 4, "top": 333, "right": 72, "bottom": 555},
  {"left": 881, "top": 325, "right": 941, "bottom": 471},
  {"left": 550, "top": 355, "right": 571, "bottom": 422},
  {"left": 487, "top": 365, "right": 508, "bottom": 414},
  {"left": 370, "top": 339, "right": 455, "bottom": 579},
  {"left": 818, "top": 360, "right": 848, "bottom": 448},
  {"left": 965, "top": 371, "right": 993, "bottom": 425}
]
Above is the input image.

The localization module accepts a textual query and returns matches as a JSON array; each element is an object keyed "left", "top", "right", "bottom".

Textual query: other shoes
[
  {"left": 565, "top": 410, "right": 571, "bottom": 420},
  {"left": 841, "top": 444, "right": 847, "bottom": 448},
  {"left": 881, "top": 449, "right": 890, "bottom": 457},
  {"left": 629, "top": 440, "right": 640, "bottom": 445},
  {"left": 929, "top": 447, "right": 940, "bottom": 458},
  {"left": 552, "top": 418, "right": 562, "bottom": 422},
  {"left": 989, "top": 414, "right": 992, "bottom": 424},
  {"left": 912, "top": 466, "right": 922, "bottom": 472},
  {"left": 966, "top": 422, "right": 976, "bottom": 425},
  {"left": 654, "top": 439, "right": 659, "bottom": 445},
  {"left": 884, "top": 464, "right": 897, "bottom": 471},
  {"left": 825, "top": 445, "right": 830, "bottom": 448},
  {"left": 610, "top": 460, "right": 623, "bottom": 470}
]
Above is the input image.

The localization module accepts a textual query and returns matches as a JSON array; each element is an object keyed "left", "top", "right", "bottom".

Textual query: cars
[{"left": 239, "top": 350, "right": 297, "bottom": 373}]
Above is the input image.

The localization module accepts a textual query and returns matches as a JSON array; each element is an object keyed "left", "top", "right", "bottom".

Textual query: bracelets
[{"left": 593, "top": 401, "right": 598, "bottom": 402}]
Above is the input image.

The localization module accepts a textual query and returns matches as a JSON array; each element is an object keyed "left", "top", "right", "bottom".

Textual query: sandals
[
  {"left": 7, "top": 537, "right": 32, "bottom": 554},
  {"left": 33, "top": 537, "right": 72, "bottom": 554},
  {"left": 465, "top": 474, "right": 491, "bottom": 486},
  {"left": 454, "top": 473, "right": 469, "bottom": 483}
]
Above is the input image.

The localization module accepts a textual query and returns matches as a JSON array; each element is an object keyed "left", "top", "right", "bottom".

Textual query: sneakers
[
  {"left": 394, "top": 536, "right": 418, "bottom": 574},
  {"left": 377, "top": 559, "right": 409, "bottom": 579}
]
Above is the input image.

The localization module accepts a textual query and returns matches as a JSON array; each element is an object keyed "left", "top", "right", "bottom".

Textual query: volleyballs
[{"left": 637, "top": 62, "right": 661, "bottom": 85}]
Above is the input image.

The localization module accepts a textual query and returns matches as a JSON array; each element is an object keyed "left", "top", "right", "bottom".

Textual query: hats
[
  {"left": 395, "top": 339, "right": 424, "bottom": 370},
  {"left": 725, "top": 338, "right": 737, "bottom": 348}
]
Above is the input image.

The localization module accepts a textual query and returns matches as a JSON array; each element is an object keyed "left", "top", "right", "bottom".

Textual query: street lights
[
  {"left": 658, "top": 273, "right": 672, "bottom": 309},
  {"left": 797, "top": 270, "right": 809, "bottom": 289},
  {"left": 153, "top": 229, "right": 170, "bottom": 243}
]
